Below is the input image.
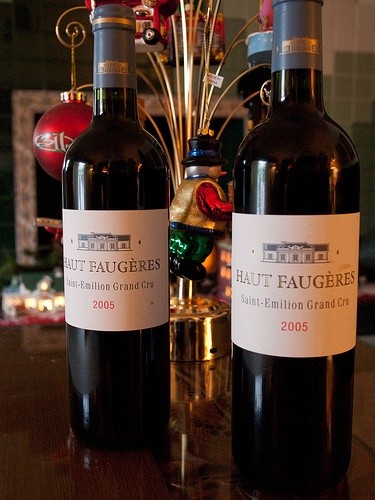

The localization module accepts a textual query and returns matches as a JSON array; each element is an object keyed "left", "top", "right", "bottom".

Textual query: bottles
[
  {"left": 228, "top": 0, "right": 361, "bottom": 500},
  {"left": 59, "top": 4, "right": 171, "bottom": 455}
]
